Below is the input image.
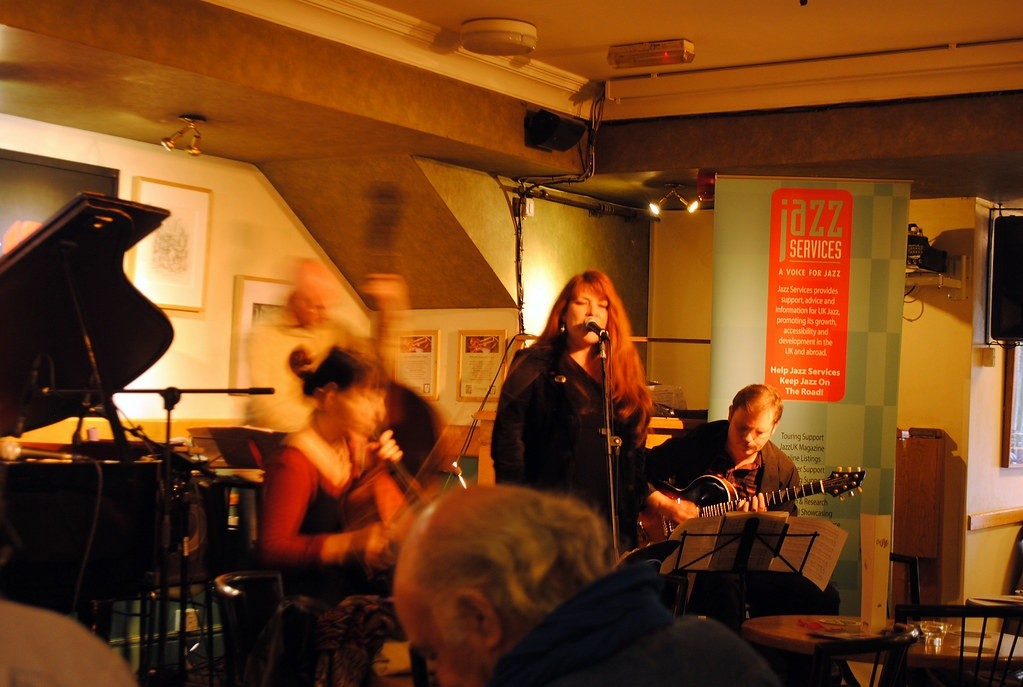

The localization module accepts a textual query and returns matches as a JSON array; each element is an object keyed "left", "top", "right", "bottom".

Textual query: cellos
[{"left": 289, "top": 344, "right": 432, "bottom": 571}]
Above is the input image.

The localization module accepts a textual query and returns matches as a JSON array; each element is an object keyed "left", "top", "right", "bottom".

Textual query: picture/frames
[
  {"left": 1002, "top": 343, "right": 1023, "bottom": 470},
  {"left": 0, "top": 147, "right": 507, "bottom": 407}
]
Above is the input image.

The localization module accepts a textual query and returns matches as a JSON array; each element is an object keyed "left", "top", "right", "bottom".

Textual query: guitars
[{"left": 638, "top": 464, "right": 868, "bottom": 550}]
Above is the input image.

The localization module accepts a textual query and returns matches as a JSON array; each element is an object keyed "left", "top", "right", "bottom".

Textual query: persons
[
  {"left": 260, "top": 344, "right": 417, "bottom": 597},
  {"left": 232, "top": 255, "right": 414, "bottom": 435},
  {"left": 489, "top": 270, "right": 653, "bottom": 553},
  {"left": 644, "top": 382, "right": 841, "bottom": 626},
  {"left": 391, "top": 481, "right": 778, "bottom": 687}
]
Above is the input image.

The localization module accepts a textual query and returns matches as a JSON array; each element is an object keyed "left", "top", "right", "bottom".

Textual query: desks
[
  {"left": 743, "top": 615, "right": 1023, "bottom": 687},
  {"left": 967, "top": 595, "right": 1023, "bottom": 608}
]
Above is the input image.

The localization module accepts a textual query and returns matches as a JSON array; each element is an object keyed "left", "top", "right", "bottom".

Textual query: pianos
[{"left": 1, "top": 186, "right": 216, "bottom": 646}]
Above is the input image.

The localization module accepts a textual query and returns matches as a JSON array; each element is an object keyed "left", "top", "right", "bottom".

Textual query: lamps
[
  {"left": 162, "top": 115, "right": 207, "bottom": 155},
  {"left": 458, "top": 19, "right": 536, "bottom": 56},
  {"left": 649, "top": 183, "right": 699, "bottom": 216},
  {"left": 608, "top": 41, "right": 696, "bottom": 70}
]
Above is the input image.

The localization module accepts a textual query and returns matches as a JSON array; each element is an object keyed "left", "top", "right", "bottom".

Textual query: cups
[{"left": 919, "top": 621, "right": 952, "bottom": 637}]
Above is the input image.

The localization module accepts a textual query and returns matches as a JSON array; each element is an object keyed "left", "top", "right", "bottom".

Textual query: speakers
[
  {"left": 991, "top": 215, "right": 1023, "bottom": 341},
  {"left": 150, "top": 480, "right": 209, "bottom": 587},
  {"left": 530, "top": 109, "right": 586, "bottom": 152}
]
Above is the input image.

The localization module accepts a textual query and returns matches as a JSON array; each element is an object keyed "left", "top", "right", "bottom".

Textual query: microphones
[
  {"left": 0, "top": 435, "right": 87, "bottom": 461},
  {"left": 585, "top": 317, "right": 610, "bottom": 342},
  {"left": 71, "top": 395, "right": 92, "bottom": 447}
]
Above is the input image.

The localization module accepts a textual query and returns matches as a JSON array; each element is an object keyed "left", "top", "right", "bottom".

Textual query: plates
[{"left": 1015, "top": 590, "right": 1023, "bottom": 595}]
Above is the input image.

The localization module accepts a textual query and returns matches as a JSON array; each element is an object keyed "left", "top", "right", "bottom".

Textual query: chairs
[
  {"left": 816, "top": 555, "right": 1023, "bottom": 687},
  {"left": 211, "top": 570, "right": 333, "bottom": 687}
]
